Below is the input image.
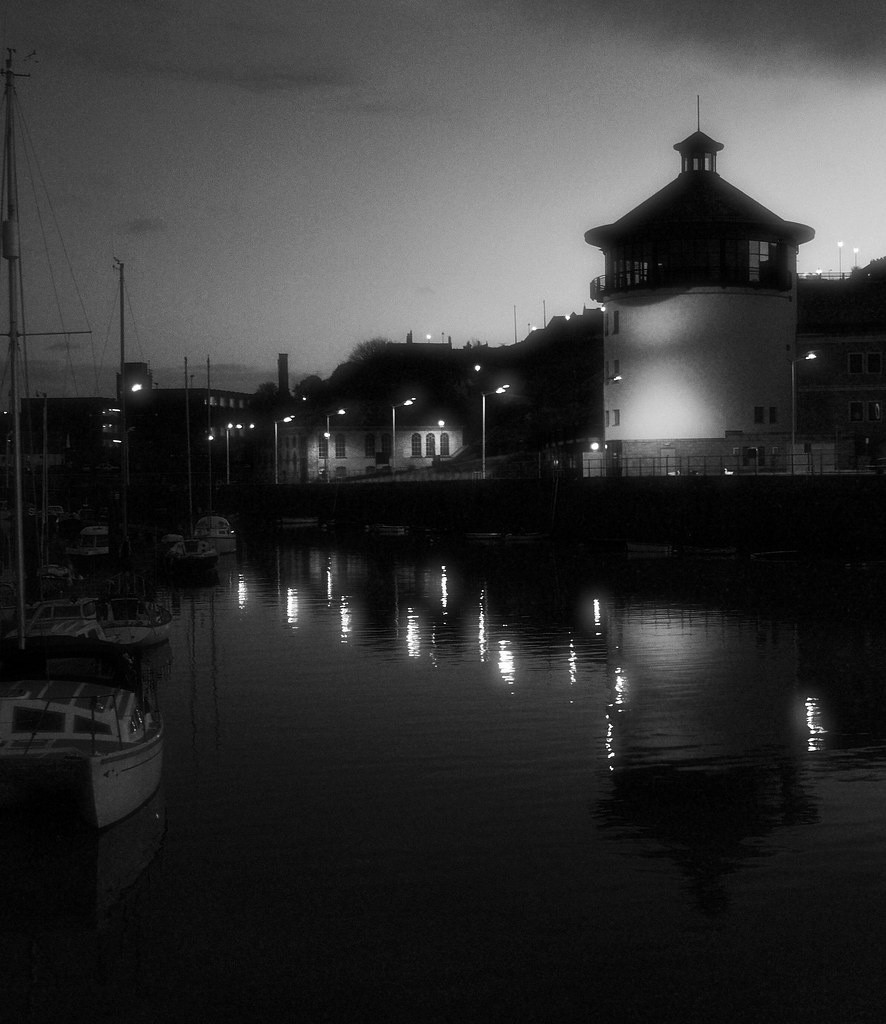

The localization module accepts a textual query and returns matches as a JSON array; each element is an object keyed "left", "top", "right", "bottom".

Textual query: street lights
[
  {"left": 275, "top": 415, "right": 295, "bottom": 485},
  {"left": 482, "top": 384, "right": 510, "bottom": 479},
  {"left": 852, "top": 246, "right": 859, "bottom": 267},
  {"left": 836, "top": 239, "right": 844, "bottom": 278},
  {"left": 226, "top": 423, "right": 232, "bottom": 485},
  {"left": 792, "top": 351, "right": 818, "bottom": 473},
  {"left": 393, "top": 398, "right": 416, "bottom": 467},
  {"left": 326, "top": 409, "right": 345, "bottom": 483}
]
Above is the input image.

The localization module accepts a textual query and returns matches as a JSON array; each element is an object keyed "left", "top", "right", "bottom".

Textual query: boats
[
  {"left": 0, "top": 47, "right": 173, "bottom": 831},
  {"left": 169, "top": 356, "right": 237, "bottom": 570}
]
